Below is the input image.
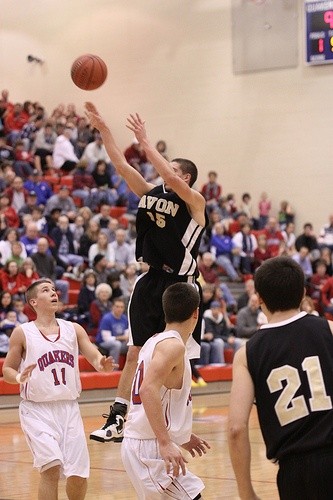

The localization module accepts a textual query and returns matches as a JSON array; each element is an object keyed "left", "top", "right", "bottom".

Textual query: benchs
[{"left": 0, "top": 153, "right": 319, "bottom": 396}]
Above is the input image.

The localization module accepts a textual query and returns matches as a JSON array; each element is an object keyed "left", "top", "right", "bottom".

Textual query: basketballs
[{"left": 70, "top": 54, "right": 109, "bottom": 92}]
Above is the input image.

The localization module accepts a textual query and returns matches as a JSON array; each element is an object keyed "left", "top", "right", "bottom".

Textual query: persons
[
  {"left": 0, "top": 277, "right": 117, "bottom": 500},
  {"left": 120, "top": 282, "right": 211, "bottom": 500},
  {"left": 0, "top": 89, "right": 170, "bottom": 371},
  {"left": 226, "top": 255, "right": 333, "bottom": 500},
  {"left": 83, "top": 101, "right": 206, "bottom": 444},
  {"left": 196, "top": 170, "right": 333, "bottom": 366}
]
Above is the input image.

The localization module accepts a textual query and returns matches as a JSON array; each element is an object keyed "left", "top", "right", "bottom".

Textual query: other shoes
[{"left": 191, "top": 375, "right": 208, "bottom": 389}]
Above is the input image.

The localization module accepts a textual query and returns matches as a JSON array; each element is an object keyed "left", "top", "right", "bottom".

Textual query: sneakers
[{"left": 90, "top": 406, "right": 127, "bottom": 444}]
